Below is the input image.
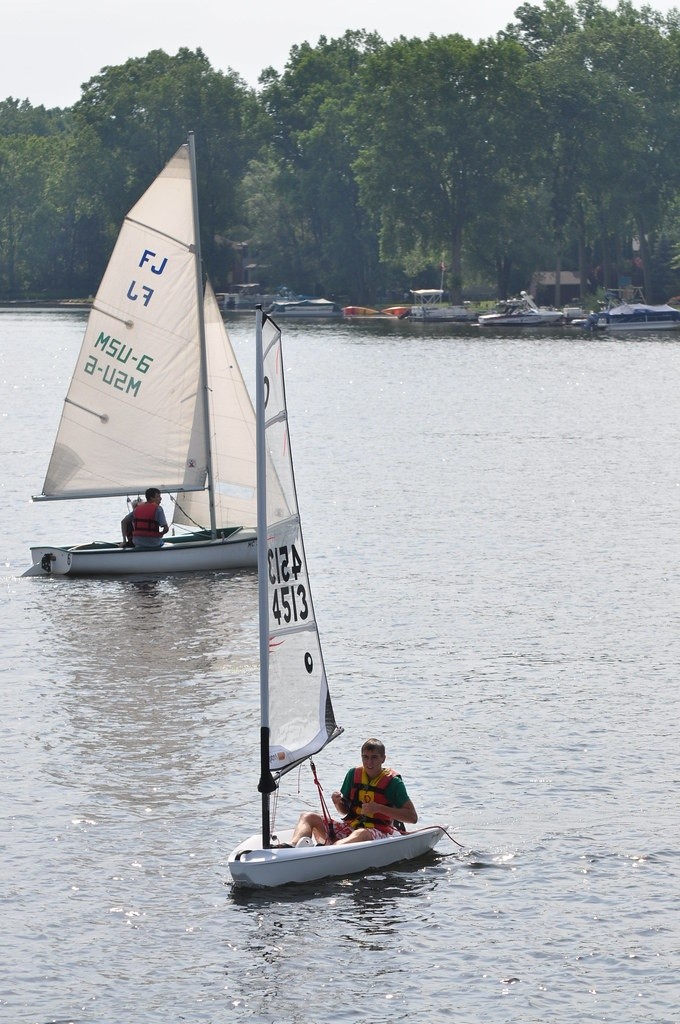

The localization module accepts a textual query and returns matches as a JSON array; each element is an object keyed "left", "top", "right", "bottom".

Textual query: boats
[
  {"left": 478, "top": 290, "right": 565, "bottom": 326},
  {"left": 595, "top": 286, "right": 680, "bottom": 332},
  {"left": 263, "top": 297, "right": 342, "bottom": 318},
  {"left": 404, "top": 288, "right": 477, "bottom": 322}
]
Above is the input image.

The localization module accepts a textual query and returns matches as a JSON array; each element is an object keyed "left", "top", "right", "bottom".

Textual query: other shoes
[
  {"left": 270, "top": 842, "right": 295, "bottom": 848},
  {"left": 316, "top": 843, "right": 326, "bottom": 846}
]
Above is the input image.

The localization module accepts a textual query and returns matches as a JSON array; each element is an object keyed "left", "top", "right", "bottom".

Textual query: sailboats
[
  {"left": 229, "top": 302, "right": 451, "bottom": 889},
  {"left": 19, "top": 127, "right": 301, "bottom": 579}
]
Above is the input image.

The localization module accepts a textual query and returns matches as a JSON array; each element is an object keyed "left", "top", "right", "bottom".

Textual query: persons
[
  {"left": 284, "top": 738, "right": 418, "bottom": 845},
  {"left": 121, "top": 488, "right": 169, "bottom": 548}
]
